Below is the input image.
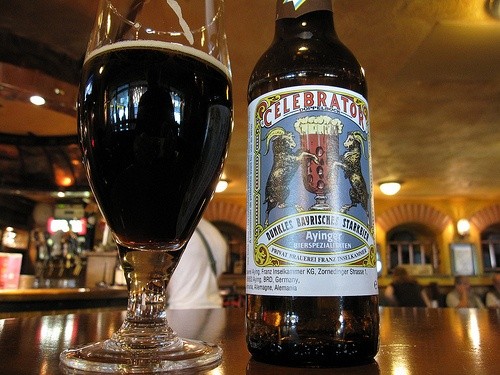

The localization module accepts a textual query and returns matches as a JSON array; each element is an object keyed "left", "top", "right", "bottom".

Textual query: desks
[{"left": 0, "top": 306, "right": 500, "bottom": 375}]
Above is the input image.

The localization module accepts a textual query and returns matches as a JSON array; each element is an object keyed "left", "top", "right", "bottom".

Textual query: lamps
[
  {"left": 378, "top": 181, "right": 403, "bottom": 196},
  {"left": 215, "top": 180, "right": 228, "bottom": 193}
]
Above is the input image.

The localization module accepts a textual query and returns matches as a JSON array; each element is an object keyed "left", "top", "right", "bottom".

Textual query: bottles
[{"left": 244, "top": 0, "right": 381, "bottom": 371}]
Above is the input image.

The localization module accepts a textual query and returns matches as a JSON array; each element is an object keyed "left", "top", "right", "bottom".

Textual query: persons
[
  {"left": 385, "top": 267, "right": 500, "bottom": 310},
  {"left": 167, "top": 218, "right": 228, "bottom": 311}
]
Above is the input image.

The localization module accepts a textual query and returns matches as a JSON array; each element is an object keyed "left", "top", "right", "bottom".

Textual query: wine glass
[{"left": 60, "top": 0, "right": 234, "bottom": 374}]
